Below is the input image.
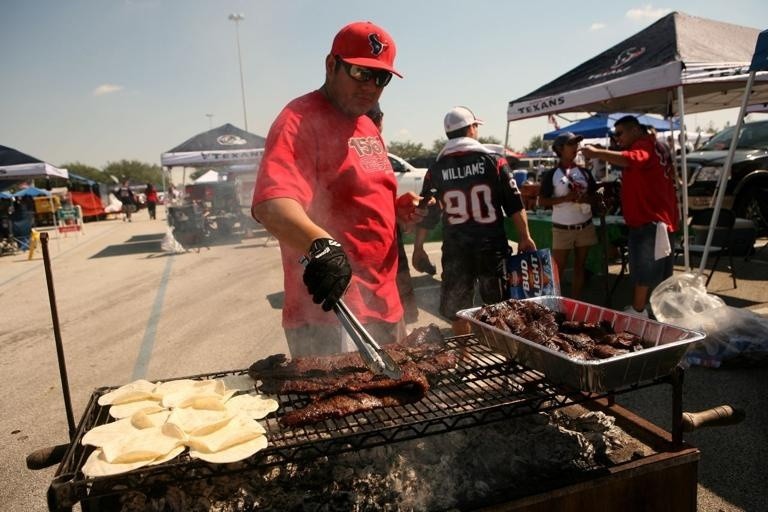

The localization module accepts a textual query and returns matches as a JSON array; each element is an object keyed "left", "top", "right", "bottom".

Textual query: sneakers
[{"left": 622, "top": 304, "right": 651, "bottom": 318}]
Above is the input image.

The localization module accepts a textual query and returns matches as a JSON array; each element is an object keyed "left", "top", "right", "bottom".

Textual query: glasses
[{"left": 341, "top": 62, "right": 393, "bottom": 89}]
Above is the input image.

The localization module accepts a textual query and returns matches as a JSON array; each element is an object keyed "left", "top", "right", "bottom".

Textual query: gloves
[
  {"left": 301, "top": 237, "right": 352, "bottom": 313},
  {"left": 412, "top": 239, "right": 431, "bottom": 273}
]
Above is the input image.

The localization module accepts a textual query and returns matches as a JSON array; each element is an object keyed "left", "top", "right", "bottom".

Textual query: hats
[
  {"left": 550, "top": 130, "right": 585, "bottom": 153},
  {"left": 328, "top": 19, "right": 405, "bottom": 77},
  {"left": 444, "top": 105, "right": 484, "bottom": 134}
]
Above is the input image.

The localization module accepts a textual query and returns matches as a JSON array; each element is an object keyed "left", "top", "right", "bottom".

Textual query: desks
[{"left": 503, "top": 209, "right": 630, "bottom": 297}]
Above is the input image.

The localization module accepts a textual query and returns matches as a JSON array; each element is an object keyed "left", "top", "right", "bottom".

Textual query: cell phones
[{"left": 416, "top": 188, "right": 438, "bottom": 209}]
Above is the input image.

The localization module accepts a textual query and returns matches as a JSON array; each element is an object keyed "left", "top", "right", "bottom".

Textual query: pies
[{"left": 81, "top": 374, "right": 279, "bottom": 477}]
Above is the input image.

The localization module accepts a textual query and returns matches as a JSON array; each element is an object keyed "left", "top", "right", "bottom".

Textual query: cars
[
  {"left": 386, "top": 152, "right": 428, "bottom": 202},
  {"left": 673, "top": 118, "right": 768, "bottom": 238}
]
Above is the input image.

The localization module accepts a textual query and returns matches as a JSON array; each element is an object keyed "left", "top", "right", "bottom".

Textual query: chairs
[{"left": 673, "top": 206, "right": 737, "bottom": 292}]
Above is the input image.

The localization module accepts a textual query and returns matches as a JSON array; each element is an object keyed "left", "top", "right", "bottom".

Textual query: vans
[{"left": 517, "top": 157, "right": 560, "bottom": 172}]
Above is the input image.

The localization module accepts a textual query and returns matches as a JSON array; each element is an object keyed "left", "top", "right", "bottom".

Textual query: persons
[
  {"left": 118, "top": 179, "right": 138, "bottom": 222},
  {"left": 573, "top": 115, "right": 681, "bottom": 320},
  {"left": 412, "top": 106, "right": 561, "bottom": 382},
  {"left": 539, "top": 132, "right": 602, "bottom": 300},
  {"left": 249, "top": 22, "right": 440, "bottom": 423},
  {"left": 588, "top": 132, "right": 694, "bottom": 181},
  {"left": 144, "top": 182, "right": 160, "bottom": 220}
]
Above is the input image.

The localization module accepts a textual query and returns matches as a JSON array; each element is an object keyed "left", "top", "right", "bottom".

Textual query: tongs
[{"left": 298, "top": 255, "right": 403, "bottom": 385}]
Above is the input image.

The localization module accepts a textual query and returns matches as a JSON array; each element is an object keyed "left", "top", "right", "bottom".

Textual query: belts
[{"left": 551, "top": 218, "right": 595, "bottom": 233}]
